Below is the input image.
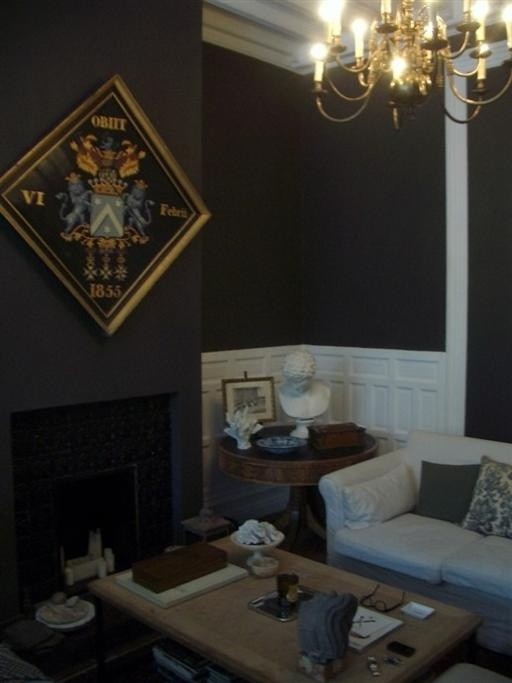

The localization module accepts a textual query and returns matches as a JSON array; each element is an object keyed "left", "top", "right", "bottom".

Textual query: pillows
[
  {"left": 417, "top": 460, "right": 482, "bottom": 524},
  {"left": 344, "top": 462, "right": 419, "bottom": 530},
  {"left": 464, "top": 455, "right": 512, "bottom": 537}
]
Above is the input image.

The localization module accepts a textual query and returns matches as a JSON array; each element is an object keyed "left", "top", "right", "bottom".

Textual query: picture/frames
[
  {"left": 0, "top": 73, "right": 213, "bottom": 339},
  {"left": 222, "top": 375, "right": 276, "bottom": 424}
]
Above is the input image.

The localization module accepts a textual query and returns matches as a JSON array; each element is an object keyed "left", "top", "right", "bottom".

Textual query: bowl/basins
[{"left": 251, "top": 556, "right": 278, "bottom": 576}]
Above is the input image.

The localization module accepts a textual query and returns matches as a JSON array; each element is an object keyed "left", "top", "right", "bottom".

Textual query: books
[
  {"left": 115, "top": 560, "right": 247, "bottom": 608},
  {"left": 345, "top": 605, "right": 403, "bottom": 650}
]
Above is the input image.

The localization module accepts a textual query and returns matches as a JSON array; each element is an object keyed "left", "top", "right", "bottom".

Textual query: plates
[
  {"left": 36, "top": 601, "right": 96, "bottom": 630},
  {"left": 255, "top": 436, "right": 308, "bottom": 454},
  {"left": 231, "top": 528, "right": 285, "bottom": 566}
]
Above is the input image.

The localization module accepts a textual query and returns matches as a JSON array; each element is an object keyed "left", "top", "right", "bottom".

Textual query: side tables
[{"left": 217, "top": 424, "right": 378, "bottom": 552}]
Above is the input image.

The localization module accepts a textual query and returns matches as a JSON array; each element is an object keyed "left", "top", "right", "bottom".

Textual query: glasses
[{"left": 359, "top": 583, "right": 405, "bottom": 613}]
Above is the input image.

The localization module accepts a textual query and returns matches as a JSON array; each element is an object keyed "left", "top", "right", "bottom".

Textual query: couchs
[{"left": 319, "top": 430, "right": 512, "bottom": 659}]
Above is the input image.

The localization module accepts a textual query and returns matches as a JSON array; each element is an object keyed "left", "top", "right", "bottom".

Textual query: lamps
[{"left": 308, "top": 0, "right": 512, "bottom": 130}]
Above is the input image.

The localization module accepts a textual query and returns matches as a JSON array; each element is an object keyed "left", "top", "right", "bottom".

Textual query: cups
[{"left": 275, "top": 573, "right": 300, "bottom": 605}]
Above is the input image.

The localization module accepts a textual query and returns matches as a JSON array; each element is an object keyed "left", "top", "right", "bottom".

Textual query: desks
[{"left": 0, "top": 578, "right": 165, "bottom": 681}]
